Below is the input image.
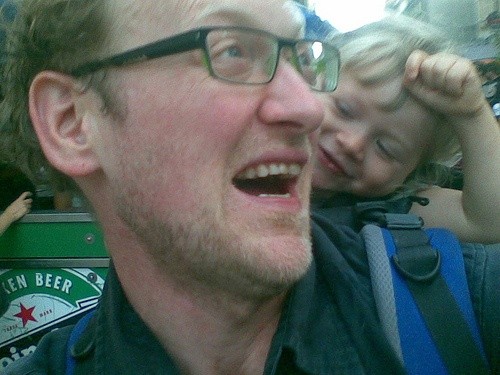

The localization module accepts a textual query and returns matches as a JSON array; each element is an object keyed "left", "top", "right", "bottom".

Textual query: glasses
[{"left": 67, "top": 26, "right": 341, "bottom": 92}]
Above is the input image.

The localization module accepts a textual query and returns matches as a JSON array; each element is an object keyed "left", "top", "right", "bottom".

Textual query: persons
[
  {"left": 0, "top": 192, "right": 32, "bottom": 237},
  {"left": 1, "top": 0, "right": 500, "bottom": 372},
  {"left": 311, "top": 18, "right": 500, "bottom": 244},
  {"left": 469, "top": 44, "right": 500, "bottom": 94}
]
